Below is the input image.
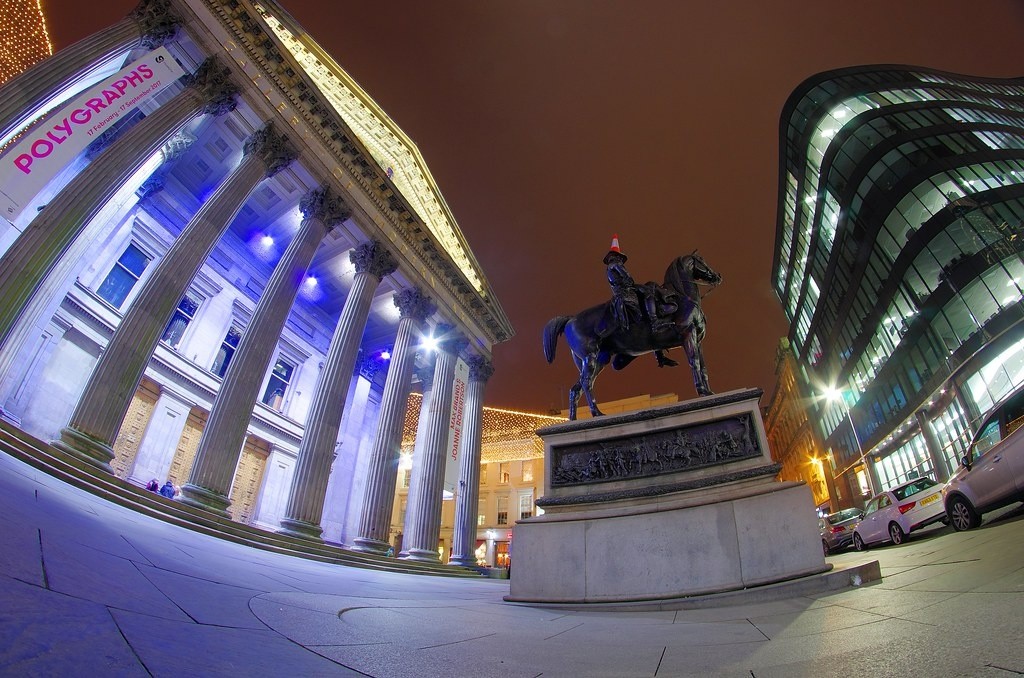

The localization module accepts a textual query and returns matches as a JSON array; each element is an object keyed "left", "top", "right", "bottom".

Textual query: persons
[
  {"left": 551, "top": 431, "right": 741, "bottom": 484},
  {"left": 589, "top": 250, "right": 680, "bottom": 368},
  {"left": 146, "top": 478, "right": 181, "bottom": 499}
]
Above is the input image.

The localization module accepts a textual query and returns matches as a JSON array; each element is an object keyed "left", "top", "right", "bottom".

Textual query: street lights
[{"left": 823, "top": 386, "right": 875, "bottom": 499}]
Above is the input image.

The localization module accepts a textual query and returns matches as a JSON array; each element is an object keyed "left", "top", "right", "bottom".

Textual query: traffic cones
[{"left": 603, "top": 231, "right": 628, "bottom": 265}]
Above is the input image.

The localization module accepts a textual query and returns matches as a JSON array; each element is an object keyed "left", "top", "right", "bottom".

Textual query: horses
[{"left": 542, "top": 248, "right": 722, "bottom": 422}]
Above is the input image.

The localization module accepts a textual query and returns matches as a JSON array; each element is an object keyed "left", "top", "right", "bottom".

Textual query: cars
[
  {"left": 940, "top": 382, "right": 1024, "bottom": 531},
  {"left": 819, "top": 507, "right": 864, "bottom": 557},
  {"left": 851, "top": 477, "right": 951, "bottom": 551}
]
